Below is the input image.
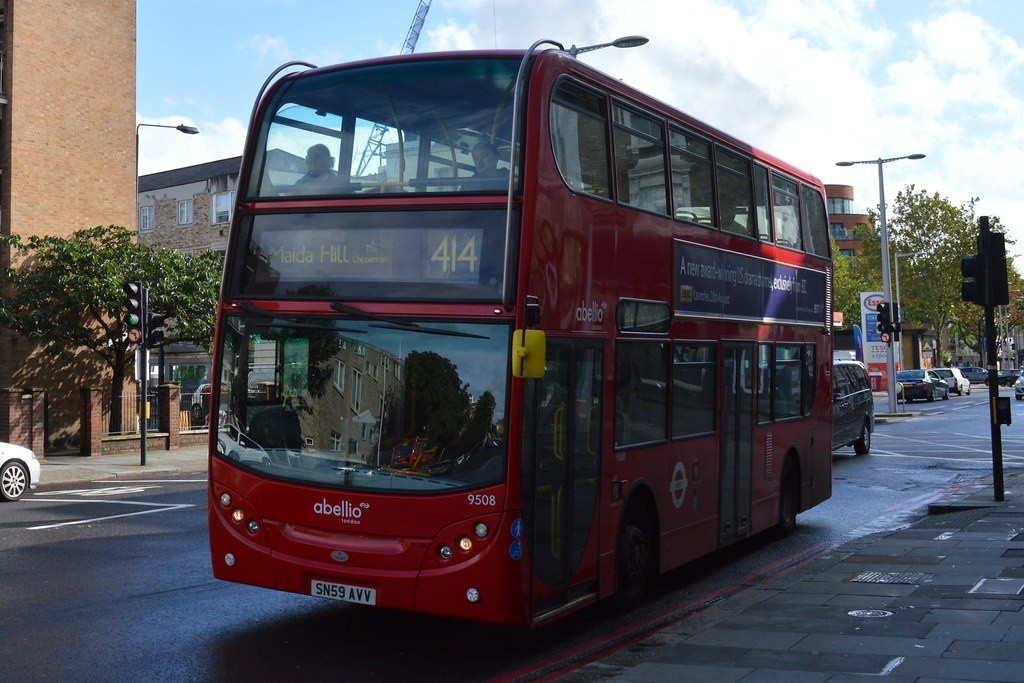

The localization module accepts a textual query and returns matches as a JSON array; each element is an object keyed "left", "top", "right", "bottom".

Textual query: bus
[
  {"left": 207, "top": 36, "right": 834, "bottom": 621},
  {"left": 388, "top": 432, "right": 478, "bottom": 476},
  {"left": 720, "top": 328, "right": 863, "bottom": 393}
]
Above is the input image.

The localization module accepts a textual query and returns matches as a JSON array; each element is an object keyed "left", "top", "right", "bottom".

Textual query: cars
[
  {"left": 1015, "top": 370, "right": 1024, "bottom": 400},
  {"left": 930, "top": 368, "right": 972, "bottom": 396},
  {"left": 216, "top": 423, "right": 272, "bottom": 470},
  {"left": 191, "top": 383, "right": 212, "bottom": 418},
  {"left": 890, "top": 370, "right": 950, "bottom": 401},
  {"left": 997, "top": 369, "right": 1021, "bottom": 388},
  {"left": 0, "top": 441, "right": 42, "bottom": 500}
]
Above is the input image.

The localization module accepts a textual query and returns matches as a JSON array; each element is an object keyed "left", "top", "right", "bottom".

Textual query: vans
[
  {"left": 832, "top": 360, "right": 875, "bottom": 456},
  {"left": 960, "top": 366, "right": 990, "bottom": 387}
]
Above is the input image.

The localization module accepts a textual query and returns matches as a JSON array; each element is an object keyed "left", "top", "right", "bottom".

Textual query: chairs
[{"left": 619, "top": 365, "right": 713, "bottom": 443}]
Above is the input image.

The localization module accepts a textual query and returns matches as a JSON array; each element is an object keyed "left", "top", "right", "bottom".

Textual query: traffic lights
[
  {"left": 962, "top": 255, "right": 984, "bottom": 307},
  {"left": 877, "top": 302, "right": 896, "bottom": 330},
  {"left": 149, "top": 313, "right": 165, "bottom": 346},
  {"left": 123, "top": 282, "right": 143, "bottom": 344}
]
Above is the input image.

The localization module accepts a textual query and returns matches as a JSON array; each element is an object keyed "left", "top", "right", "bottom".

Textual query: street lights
[
  {"left": 836, "top": 152, "right": 928, "bottom": 411},
  {"left": 134, "top": 123, "right": 201, "bottom": 194},
  {"left": 895, "top": 251, "right": 936, "bottom": 411},
  {"left": 572, "top": 34, "right": 649, "bottom": 58},
  {"left": 378, "top": 355, "right": 404, "bottom": 466}
]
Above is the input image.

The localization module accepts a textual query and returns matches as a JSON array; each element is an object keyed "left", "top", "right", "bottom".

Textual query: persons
[
  {"left": 459, "top": 142, "right": 510, "bottom": 190},
  {"left": 295, "top": 144, "right": 336, "bottom": 186},
  {"left": 720, "top": 198, "right": 746, "bottom": 234}
]
